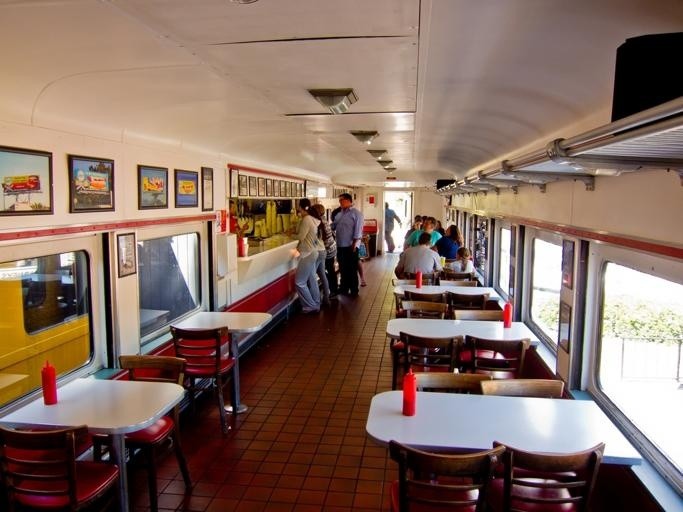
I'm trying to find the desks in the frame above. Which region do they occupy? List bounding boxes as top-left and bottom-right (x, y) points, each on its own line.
(174, 311), (273, 415)
(0, 376), (185, 511)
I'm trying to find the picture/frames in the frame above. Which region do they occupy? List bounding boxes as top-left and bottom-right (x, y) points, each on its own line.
(227, 168), (240, 199)
(199, 166), (214, 211)
(117, 232), (137, 278)
(136, 165), (168, 210)
(238, 175), (306, 200)
(0, 145), (54, 216)
(68, 154), (115, 213)
(174, 169), (198, 208)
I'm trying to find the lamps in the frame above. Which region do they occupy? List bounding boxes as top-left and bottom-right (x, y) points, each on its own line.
(367, 149), (388, 161)
(306, 88), (361, 117)
(383, 167), (397, 173)
(349, 130), (380, 146)
(376, 161), (394, 169)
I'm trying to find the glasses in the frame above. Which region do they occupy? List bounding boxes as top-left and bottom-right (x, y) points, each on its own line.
(339, 199), (344, 202)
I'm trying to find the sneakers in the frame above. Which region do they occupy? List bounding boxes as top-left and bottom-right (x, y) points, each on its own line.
(328, 292), (337, 298)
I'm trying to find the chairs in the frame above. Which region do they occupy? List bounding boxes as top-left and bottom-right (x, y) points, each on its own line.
(0, 424), (120, 511)
(92, 355), (194, 511)
(168, 324), (238, 435)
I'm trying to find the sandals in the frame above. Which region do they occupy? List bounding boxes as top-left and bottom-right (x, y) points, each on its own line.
(361, 280), (366, 287)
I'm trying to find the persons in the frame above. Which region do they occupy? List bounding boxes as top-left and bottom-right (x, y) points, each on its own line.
(286, 198), (321, 313)
(229, 200), (249, 240)
(395, 253), (475, 281)
(331, 206), (367, 287)
(309, 207), (331, 306)
(330, 193), (364, 301)
(385, 202), (403, 253)
(312, 204), (338, 300)
(402, 214), (471, 253)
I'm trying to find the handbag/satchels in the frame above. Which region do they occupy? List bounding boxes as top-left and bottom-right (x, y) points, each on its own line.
(358, 243), (366, 260)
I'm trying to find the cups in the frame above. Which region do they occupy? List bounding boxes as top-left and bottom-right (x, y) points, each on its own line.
(440, 257), (445, 268)
(254, 218), (267, 240)
(265, 200), (282, 235)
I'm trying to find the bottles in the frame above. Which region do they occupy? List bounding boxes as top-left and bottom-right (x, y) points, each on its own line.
(503, 302), (511, 328)
(415, 272), (421, 288)
(238, 234), (244, 257)
(401, 368), (416, 417)
(41, 360), (57, 406)
(243, 237), (248, 256)
